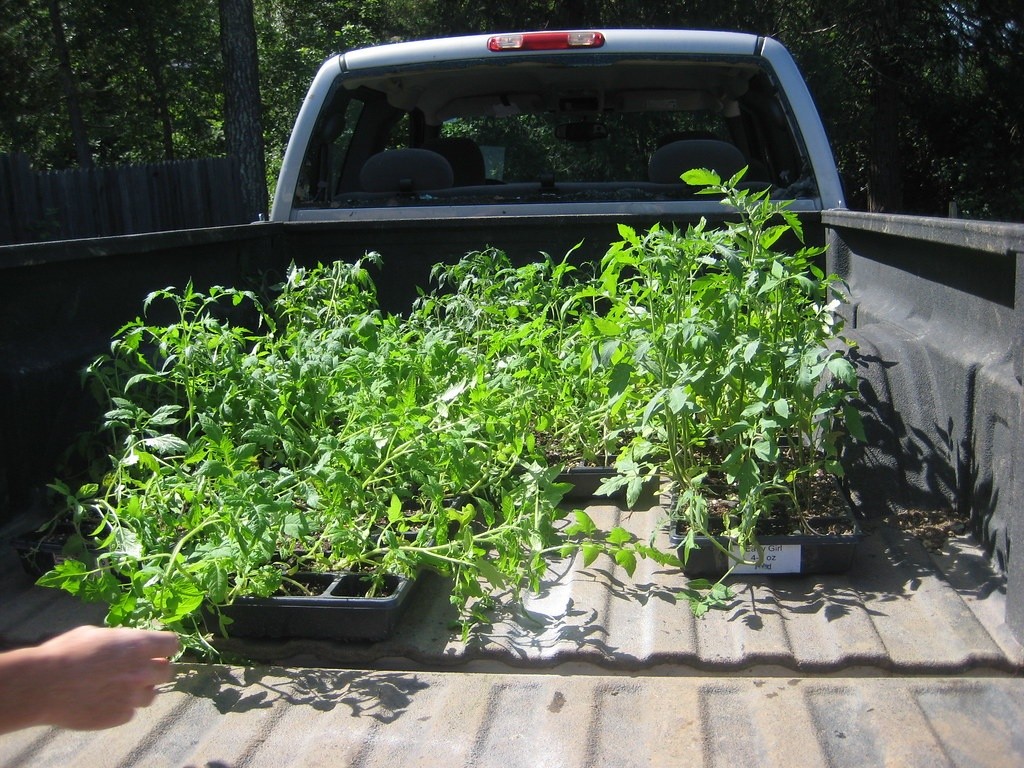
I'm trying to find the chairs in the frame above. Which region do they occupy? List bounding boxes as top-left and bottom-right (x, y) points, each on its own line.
(363, 149), (455, 195)
(419, 138), (506, 187)
(650, 138), (747, 185)
(657, 130), (715, 147)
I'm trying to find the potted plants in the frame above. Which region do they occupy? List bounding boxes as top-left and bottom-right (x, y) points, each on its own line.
(566, 165), (862, 574)
(24, 248), (686, 666)
(433, 249), (666, 501)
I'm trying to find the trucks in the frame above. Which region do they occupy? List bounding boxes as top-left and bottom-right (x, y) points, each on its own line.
(1, 28), (1024, 768)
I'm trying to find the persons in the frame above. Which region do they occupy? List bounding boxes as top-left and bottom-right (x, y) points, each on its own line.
(0, 625), (180, 735)
